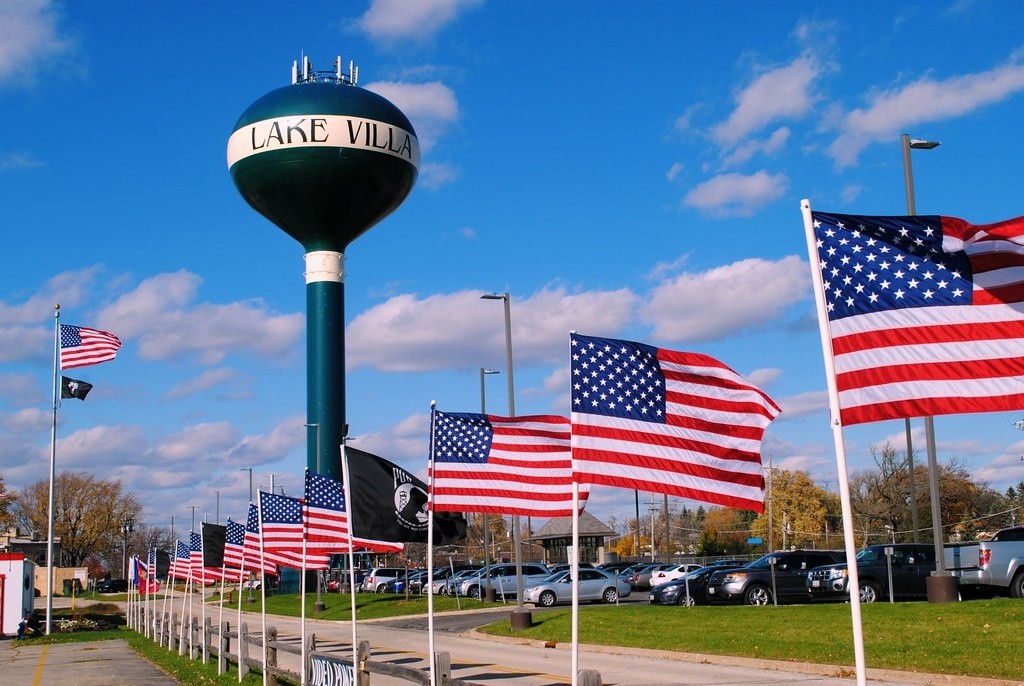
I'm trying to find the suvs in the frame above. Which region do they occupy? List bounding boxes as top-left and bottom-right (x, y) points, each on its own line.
(703, 548), (847, 608)
(804, 542), (951, 605)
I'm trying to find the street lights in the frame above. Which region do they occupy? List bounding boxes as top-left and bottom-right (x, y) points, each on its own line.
(238, 465), (252, 504)
(162, 514), (174, 550)
(901, 131), (962, 601)
(479, 367), (502, 587)
(208, 491), (222, 526)
(120, 523), (135, 579)
(481, 289), (533, 629)
(186, 505), (199, 533)
(302, 420), (322, 476)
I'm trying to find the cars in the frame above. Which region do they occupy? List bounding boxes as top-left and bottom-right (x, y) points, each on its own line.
(516, 567), (633, 607)
(648, 563), (705, 589)
(648, 565), (742, 610)
(386, 561), (552, 601)
(704, 558), (750, 568)
(325, 570), (364, 594)
(96, 579), (129, 593)
(360, 566), (413, 594)
(545, 560), (675, 590)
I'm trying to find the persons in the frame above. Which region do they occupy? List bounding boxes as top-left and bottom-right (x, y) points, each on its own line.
(354, 569), (365, 593)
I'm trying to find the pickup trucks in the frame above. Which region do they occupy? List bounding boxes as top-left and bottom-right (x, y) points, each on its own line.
(936, 523), (1024, 601)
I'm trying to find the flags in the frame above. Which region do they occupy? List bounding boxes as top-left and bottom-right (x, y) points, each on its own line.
(344, 446), (467, 546)
(260, 491), (361, 552)
(244, 504), (331, 571)
(60, 323), (123, 371)
(812, 210), (1024, 428)
(433, 410), (591, 518)
(61, 376), (93, 401)
(570, 332), (783, 515)
(130, 519), (278, 595)
(307, 472), (404, 554)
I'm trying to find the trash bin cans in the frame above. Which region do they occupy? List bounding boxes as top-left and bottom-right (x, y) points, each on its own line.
(604, 552), (617, 563)
(63, 578), (80, 596)
